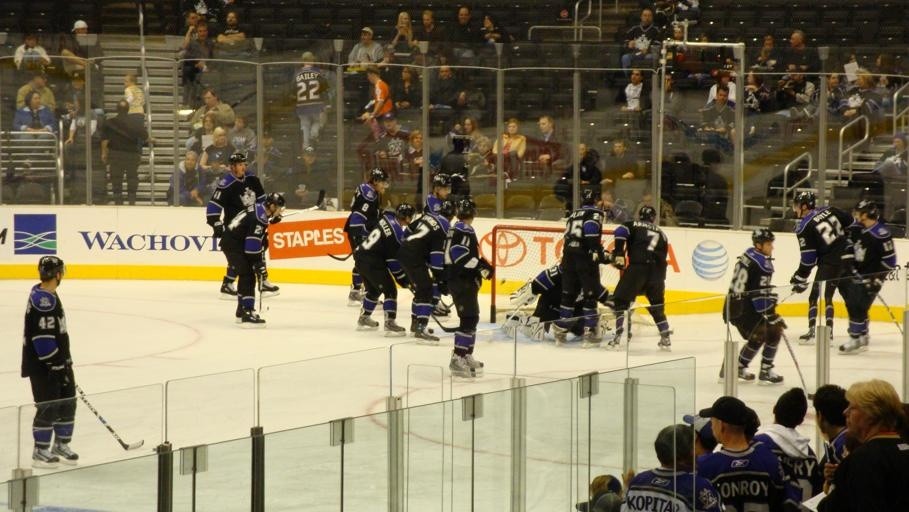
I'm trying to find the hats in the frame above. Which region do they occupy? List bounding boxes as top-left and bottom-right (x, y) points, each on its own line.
(773, 388), (807, 428)
(382, 112), (396, 122)
(302, 53), (316, 60)
(305, 146), (315, 155)
(699, 396), (748, 424)
(576, 489), (621, 512)
(71, 20), (88, 32)
(361, 27), (373, 34)
(744, 408), (761, 432)
(684, 414), (717, 448)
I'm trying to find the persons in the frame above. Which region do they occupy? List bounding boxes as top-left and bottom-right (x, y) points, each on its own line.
(825, 199), (899, 353)
(841, 52), (873, 86)
(623, 8), (665, 79)
(377, 111), (412, 169)
(652, 74), (684, 125)
(442, 9), (477, 62)
(779, 67), (816, 109)
(191, 113), (218, 156)
(477, 15), (514, 76)
(398, 208), (458, 343)
(442, 199), (493, 379)
(744, 68), (774, 112)
(401, 131), (433, 175)
(413, 11), (441, 44)
(344, 169), (390, 310)
(366, 67), (397, 137)
(705, 70), (739, 107)
(22, 256), (79, 469)
(425, 174), (454, 317)
(603, 142), (639, 189)
(15, 77), (56, 109)
(431, 64), (464, 108)
(781, 33), (822, 73)
(694, 395), (786, 510)
(169, 151), (209, 206)
(10, 93), (57, 170)
(63, 92), (98, 185)
(347, 26), (385, 78)
(661, 24), (691, 67)
(493, 118), (527, 183)
(290, 51), (329, 155)
(355, 202), (415, 338)
(874, 133), (909, 185)
(867, 51), (903, 88)
(624, 424), (725, 512)
(182, 21), (216, 108)
(247, 135), (283, 171)
(177, 11), (201, 35)
(289, 147), (329, 202)
(839, 72), (884, 127)
(220, 194), (285, 327)
(699, 87), (737, 138)
(528, 249), (613, 335)
(14, 31), (52, 82)
(585, 470), (634, 501)
(607, 204), (674, 349)
(751, 385), (822, 510)
(679, 411), (718, 462)
(790, 190), (867, 345)
(686, 34), (722, 74)
(544, 199), (607, 346)
(752, 35), (784, 74)
(813, 384), (851, 481)
(118, 72), (146, 151)
(190, 87), (236, 131)
(465, 137), (501, 178)
(388, 12), (418, 69)
(557, 145), (603, 206)
(817, 380), (909, 512)
(205, 154), (282, 295)
(218, 10), (252, 71)
(689, 150), (730, 225)
(618, 69), (654, 133)
(62, 19), (105, 108)
(530, 117), (565, 166)
(724, 229), (787, 383)
(100, 99), (155, 208)
(449, 117), (482, 155)
(62, 72), (97, 118)
(393, 65), (426, 111)
(226, 113), (257, 156)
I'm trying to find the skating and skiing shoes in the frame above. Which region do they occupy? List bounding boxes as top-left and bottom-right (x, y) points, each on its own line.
(830, 334), (834, 348)
(348, 290), (364, 306)
(258, 281), (279, 298)
(374, 300), (383, 310)
(435, 306), (451, 318)
(429, 310), (448, 321)
(658, 336), (672, 352)
(384, 321), (406, 337)
(32, 448), (60, 469)
(555, 334), (572, 347)
(357, 317), (380, 330)
(582, 335), (602, 349)
(837, 339), (862, 355)
(235, 306), (260, 323)
(860, 336), (869, 350)
(51, 442), (79, 465)
(220, 283), (239, 301)
(799, 331), (816, 345)
(411, 326), (434, 338)
(738, 367), (755, 383)
(450, 358), (475, 382)
(607, 335), (631, 351)
(242, 312), (266, 329)
(758, 371), (784, 385)
(415, 331), (439, 346)
(464, 355), (484, 378)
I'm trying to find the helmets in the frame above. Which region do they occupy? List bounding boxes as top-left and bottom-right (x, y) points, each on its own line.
(752, 229), (774, 243)
(229, 153), (247, 165)
(794, 192), (816, 209)
(433, 174), (452, 187)
(266, 192), (284, 208)
(39, 256), (63, 280)
(439, 201), (457, 217)
(855, 199), (880, 222)
(640, 206), (656, 222)
(583, 186), (601, 201)
(396, 204), (414, 218)
(370, 168), (387, 182)
(456, 199), (475, 218)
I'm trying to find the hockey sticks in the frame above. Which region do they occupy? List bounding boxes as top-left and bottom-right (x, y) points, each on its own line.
(875, 292), (904, 334)
(408, 284), (460, 333)
(259, 275), (270, 314)
(776, 290), (797, 306)
(439, 297), (455, 309)
(780, 326), (817, 400)
(75, 383), (145, 451)
(327, 251), (353, 261)
(267, 189), (326, 222)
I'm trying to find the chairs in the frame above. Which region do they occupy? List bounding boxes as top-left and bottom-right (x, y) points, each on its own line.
(154, 0), (909, 239)
(0, 0), (108, 205)
